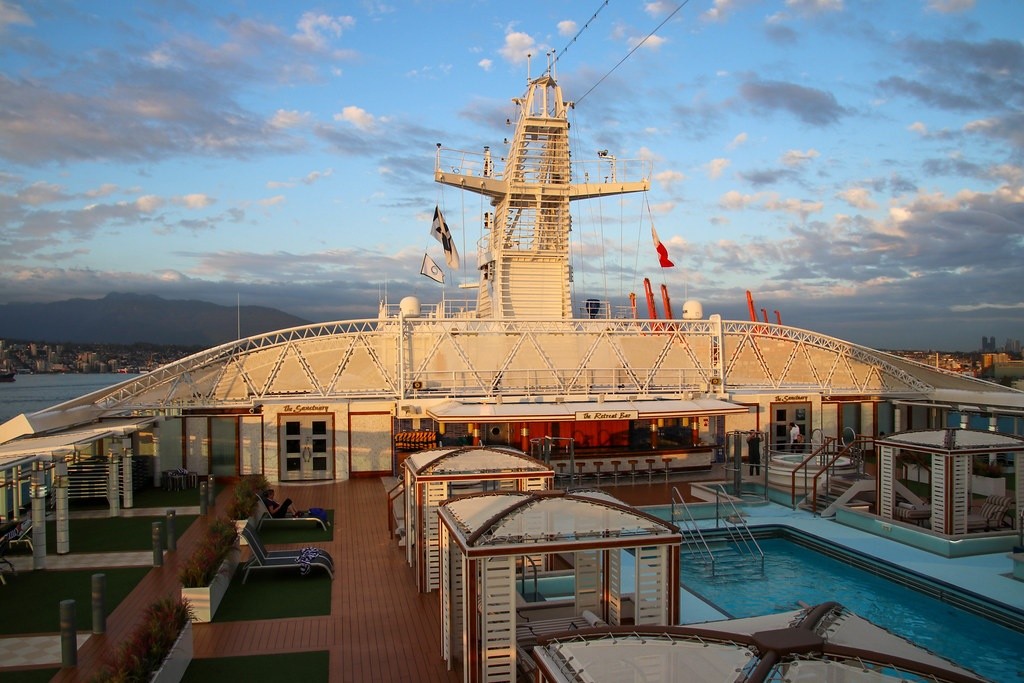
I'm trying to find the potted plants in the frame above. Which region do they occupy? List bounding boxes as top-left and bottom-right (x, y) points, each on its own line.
(227, 473), (273, 546)
(175, 512), (241, 623)
(86, 592), (193, 682)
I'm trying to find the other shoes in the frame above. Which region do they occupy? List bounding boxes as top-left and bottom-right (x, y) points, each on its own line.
(292, 511), (303, 518)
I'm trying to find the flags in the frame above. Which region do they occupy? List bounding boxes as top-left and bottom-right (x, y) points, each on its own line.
(430, 206), (461, 271)
(420, 253), (446, 285)
(651, 223), (675, 268)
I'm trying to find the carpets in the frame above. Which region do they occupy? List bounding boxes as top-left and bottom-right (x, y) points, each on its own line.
(0, 564), (155, 638)
(45, 475), (226, 511)
(0, 514), (199, 557)
(211, 561), (331, 622)
(0, 663), (62, 683)
(257, 508), (335, 544)
(180, 650), (329, 683)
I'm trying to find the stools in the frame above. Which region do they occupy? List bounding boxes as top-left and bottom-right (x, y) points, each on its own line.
(162, 470), (198, 491)
(593, 462), (604, 485)
(644, 459), (656, 482)
(661, 459), (672, 481)
(611, 460), (622, 484)
(628, 460), (639, 483)
(575, 462), (586, 486)
(556, 463), (566, 486)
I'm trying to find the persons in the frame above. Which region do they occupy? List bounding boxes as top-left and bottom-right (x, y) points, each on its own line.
(789, 422), (803, 453)
(264, 489), (304, 518)
(746, 430), (765, 476)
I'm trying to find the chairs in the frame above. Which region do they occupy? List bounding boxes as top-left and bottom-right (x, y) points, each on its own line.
(50, 455), (153, 509)
(491, 610), (611, 683)
(893, 495), (1014, 532)
(255, 489), (331, 532)
(241, 522), (335, 580)
(8, 525), (33, 552)
